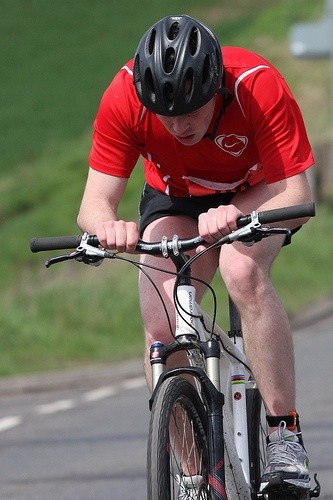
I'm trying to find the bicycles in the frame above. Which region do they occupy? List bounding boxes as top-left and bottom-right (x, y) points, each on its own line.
(30, 201), (321, 500)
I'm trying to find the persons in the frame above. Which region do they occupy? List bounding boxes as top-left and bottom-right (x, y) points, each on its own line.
(77, 15), (315, 500)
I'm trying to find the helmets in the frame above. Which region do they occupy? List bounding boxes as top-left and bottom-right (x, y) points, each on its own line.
(131, 15), (221, 117)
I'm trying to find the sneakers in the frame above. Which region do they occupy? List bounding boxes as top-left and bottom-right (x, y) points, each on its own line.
(260, 413), (322, 498)
(175, 465), (207, 500)
